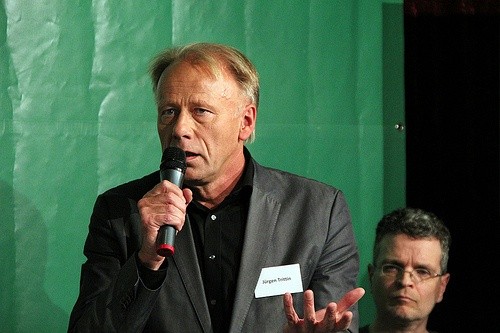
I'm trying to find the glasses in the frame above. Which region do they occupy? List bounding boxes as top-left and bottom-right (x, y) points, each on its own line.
(376, 263), (444, 282)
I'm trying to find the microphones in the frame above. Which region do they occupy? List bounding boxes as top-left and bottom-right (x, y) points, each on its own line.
(157, 147), (187, 257)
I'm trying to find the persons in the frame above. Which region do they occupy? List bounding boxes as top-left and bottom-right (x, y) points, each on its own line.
(358, 207), (452, 333)
(67, 40), (367, 333)
